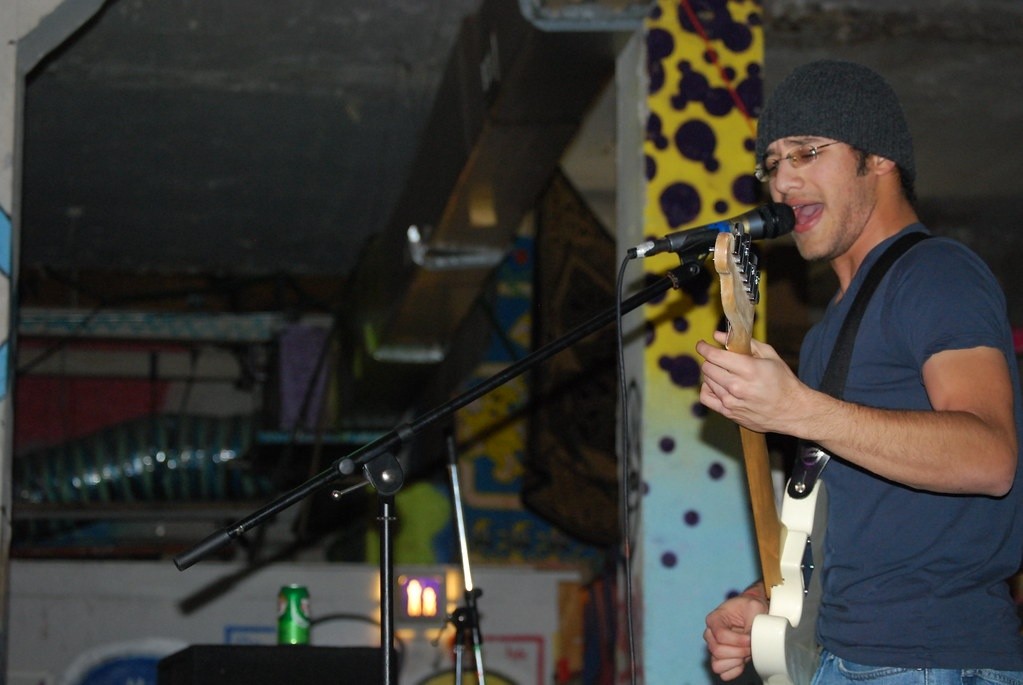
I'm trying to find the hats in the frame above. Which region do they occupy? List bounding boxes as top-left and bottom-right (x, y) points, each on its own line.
(754, 56), (917, 176)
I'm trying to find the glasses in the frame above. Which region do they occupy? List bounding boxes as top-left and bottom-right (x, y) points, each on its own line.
(757, 138), (842, 182)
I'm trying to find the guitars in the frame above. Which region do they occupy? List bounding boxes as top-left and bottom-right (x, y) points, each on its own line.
(710, 221), (829, 685)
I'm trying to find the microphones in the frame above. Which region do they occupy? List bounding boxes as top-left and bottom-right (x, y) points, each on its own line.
(626, 201), (794, 260)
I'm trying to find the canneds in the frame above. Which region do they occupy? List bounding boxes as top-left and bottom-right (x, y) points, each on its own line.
(277, 583), (311, 646)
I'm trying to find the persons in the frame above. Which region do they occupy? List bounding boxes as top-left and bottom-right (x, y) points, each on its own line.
(696, 60), (1023, 685)
